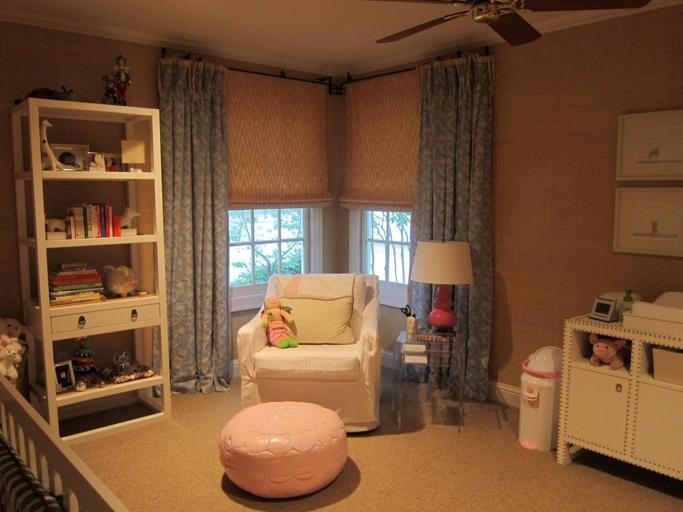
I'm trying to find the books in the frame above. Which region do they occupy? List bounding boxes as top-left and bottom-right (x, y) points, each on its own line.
(48, 260), (104, 305)
(66, 199), (122, 239)
(416, 332), (453, 342)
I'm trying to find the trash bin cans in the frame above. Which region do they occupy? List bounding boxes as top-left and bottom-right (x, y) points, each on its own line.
(519, 346), (571, 450)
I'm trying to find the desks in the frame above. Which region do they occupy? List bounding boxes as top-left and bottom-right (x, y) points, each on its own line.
(393, 331), (472, 433)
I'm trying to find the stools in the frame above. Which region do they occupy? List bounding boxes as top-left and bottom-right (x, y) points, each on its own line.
(220, 401), (348, 499)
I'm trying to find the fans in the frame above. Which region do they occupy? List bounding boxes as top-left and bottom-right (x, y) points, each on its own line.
(376, 0), (653, 47)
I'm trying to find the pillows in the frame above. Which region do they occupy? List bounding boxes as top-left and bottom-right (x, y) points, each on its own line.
(280, 296), (356, 345)
(278, 274), (366, 339)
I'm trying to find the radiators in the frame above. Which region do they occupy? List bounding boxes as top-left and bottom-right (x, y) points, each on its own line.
(0, 431), (64, 512)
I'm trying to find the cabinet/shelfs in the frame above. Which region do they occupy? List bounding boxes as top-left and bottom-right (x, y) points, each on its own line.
(556, 313), (683, 481)
(10, 97), (172, 442)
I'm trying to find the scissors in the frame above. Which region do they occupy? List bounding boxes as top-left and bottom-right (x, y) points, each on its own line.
(401, 305), (411, 317)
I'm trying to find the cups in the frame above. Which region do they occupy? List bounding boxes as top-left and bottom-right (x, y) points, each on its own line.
(406, 316), (419, 334)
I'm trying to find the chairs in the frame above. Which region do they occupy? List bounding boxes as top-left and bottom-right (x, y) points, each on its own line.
(236, 272), (381, 433)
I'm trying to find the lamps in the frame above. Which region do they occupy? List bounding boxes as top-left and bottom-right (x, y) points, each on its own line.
(410, 241), (473, 336)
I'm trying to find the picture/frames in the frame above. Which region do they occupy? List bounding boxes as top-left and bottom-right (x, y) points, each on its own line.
(612, 187), (683, 257)
(616, 109), (683, 181)
(54, 360), (75, 393)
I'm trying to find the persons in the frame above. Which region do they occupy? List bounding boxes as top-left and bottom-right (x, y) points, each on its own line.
(102, 78), (117, 104)
(112, 55), (132, 106)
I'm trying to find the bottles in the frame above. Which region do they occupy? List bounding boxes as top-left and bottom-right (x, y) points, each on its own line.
(620, 287), (635, 324)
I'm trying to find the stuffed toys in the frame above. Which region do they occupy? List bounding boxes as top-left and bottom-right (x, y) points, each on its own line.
(0, 317), (30, 341)
(0, 335), (23, 380)
(258, 296), (298, 347)
(585, 334), (627, 371)
